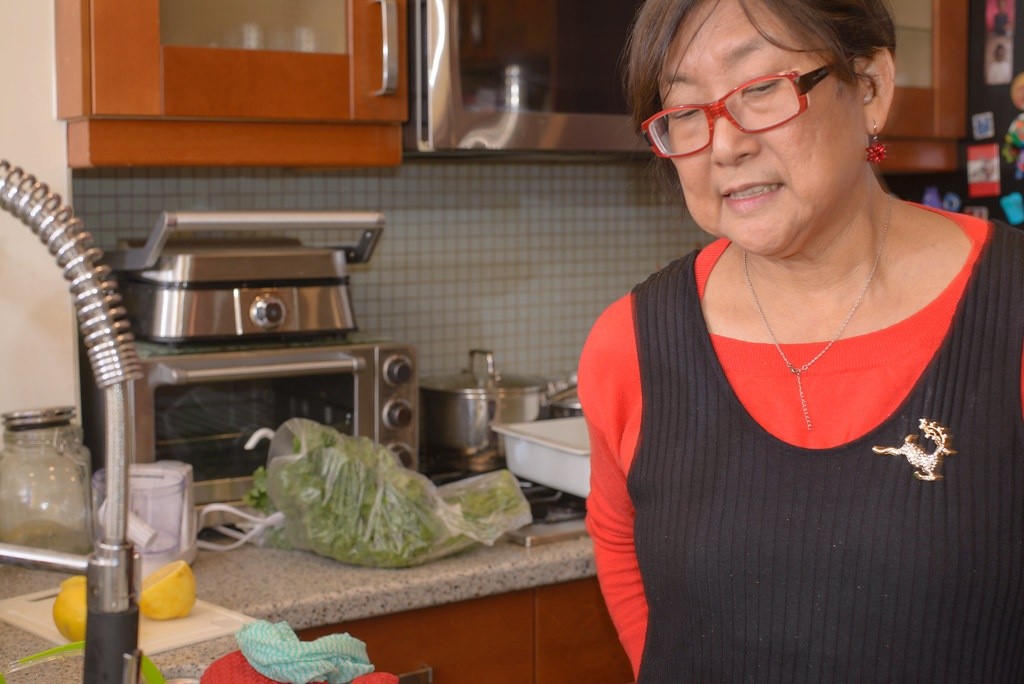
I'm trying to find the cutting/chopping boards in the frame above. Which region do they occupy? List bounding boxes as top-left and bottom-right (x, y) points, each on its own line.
(1, 589), (260, 656)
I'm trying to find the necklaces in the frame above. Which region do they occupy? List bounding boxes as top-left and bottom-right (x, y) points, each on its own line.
(739, 193), (892, 433)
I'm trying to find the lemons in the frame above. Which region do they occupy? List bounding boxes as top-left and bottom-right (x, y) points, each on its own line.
(51, 559), (194, 641)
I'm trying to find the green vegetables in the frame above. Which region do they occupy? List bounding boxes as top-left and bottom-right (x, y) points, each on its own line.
(243, 426), (525, 568)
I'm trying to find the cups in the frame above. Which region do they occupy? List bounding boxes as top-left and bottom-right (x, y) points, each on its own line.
(95, 462), (185, 581)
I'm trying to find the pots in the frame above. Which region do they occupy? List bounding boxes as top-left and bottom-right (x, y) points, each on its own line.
(551, 376), (584, 417)
(420, 348), (548, 451)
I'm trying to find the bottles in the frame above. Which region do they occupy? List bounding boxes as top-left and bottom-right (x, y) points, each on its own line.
(0, 405), (94, 555)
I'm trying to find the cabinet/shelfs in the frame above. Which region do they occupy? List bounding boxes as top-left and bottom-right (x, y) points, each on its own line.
(292, 575), (632, 684)
(51, 0), (410, 170)
(867, 1), (966, 170)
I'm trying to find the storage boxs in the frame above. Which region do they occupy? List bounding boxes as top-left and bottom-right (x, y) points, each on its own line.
(490, 412), (592, 498)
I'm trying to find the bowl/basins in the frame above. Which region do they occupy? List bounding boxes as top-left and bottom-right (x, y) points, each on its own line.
(0, 641), (167, 683)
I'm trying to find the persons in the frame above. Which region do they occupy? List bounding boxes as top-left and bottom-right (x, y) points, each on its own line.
(573, 0), (1024, 684)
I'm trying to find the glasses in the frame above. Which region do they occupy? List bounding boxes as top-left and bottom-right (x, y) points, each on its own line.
(641, 36), (878, 159)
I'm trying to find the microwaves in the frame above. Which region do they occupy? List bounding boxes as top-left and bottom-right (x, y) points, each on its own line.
(403, 0), (659, 157)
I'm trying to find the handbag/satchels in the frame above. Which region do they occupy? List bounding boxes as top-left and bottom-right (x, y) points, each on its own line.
(193, 415), (536, 571)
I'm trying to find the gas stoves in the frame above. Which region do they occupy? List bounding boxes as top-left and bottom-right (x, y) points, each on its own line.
(421, 449), (590, 549)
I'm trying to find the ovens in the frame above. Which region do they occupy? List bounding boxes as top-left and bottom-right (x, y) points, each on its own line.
(81, 210), (420, 533)
